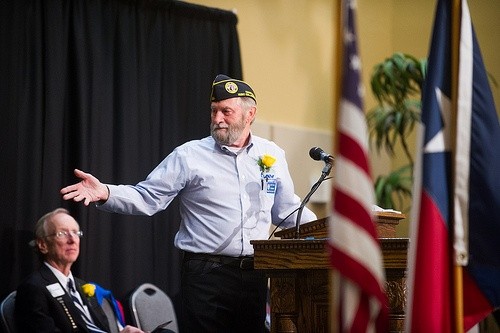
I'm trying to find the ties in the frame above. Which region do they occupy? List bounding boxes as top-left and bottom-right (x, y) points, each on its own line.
(67, 277), (109, 333)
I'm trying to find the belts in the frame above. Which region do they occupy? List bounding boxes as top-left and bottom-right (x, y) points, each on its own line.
(180, 249), (256, 270)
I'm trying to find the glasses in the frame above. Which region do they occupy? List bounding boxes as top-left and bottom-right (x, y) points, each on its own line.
(40, 229), (83, 240)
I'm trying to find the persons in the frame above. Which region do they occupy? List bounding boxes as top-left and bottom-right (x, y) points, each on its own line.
(13, 209), (152, 333)
(60, 74), (319, 333)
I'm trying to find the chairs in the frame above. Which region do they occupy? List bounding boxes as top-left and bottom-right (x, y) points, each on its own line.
(130, 282), (178, 333)
(2, 291), (18, 333)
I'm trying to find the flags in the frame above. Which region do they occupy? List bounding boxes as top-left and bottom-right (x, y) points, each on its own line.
(405, 0), (500, 333)
(328, 0), (388, 333)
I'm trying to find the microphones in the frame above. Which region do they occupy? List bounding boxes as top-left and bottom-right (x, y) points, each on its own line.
(309, 147), (334, 163)
(68, 279), (75, 293)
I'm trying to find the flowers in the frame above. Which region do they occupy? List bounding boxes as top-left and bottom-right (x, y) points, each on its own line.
(257, 155), (276, 171)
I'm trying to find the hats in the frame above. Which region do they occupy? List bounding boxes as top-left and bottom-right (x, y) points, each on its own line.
(210, 74), (258, 102)
